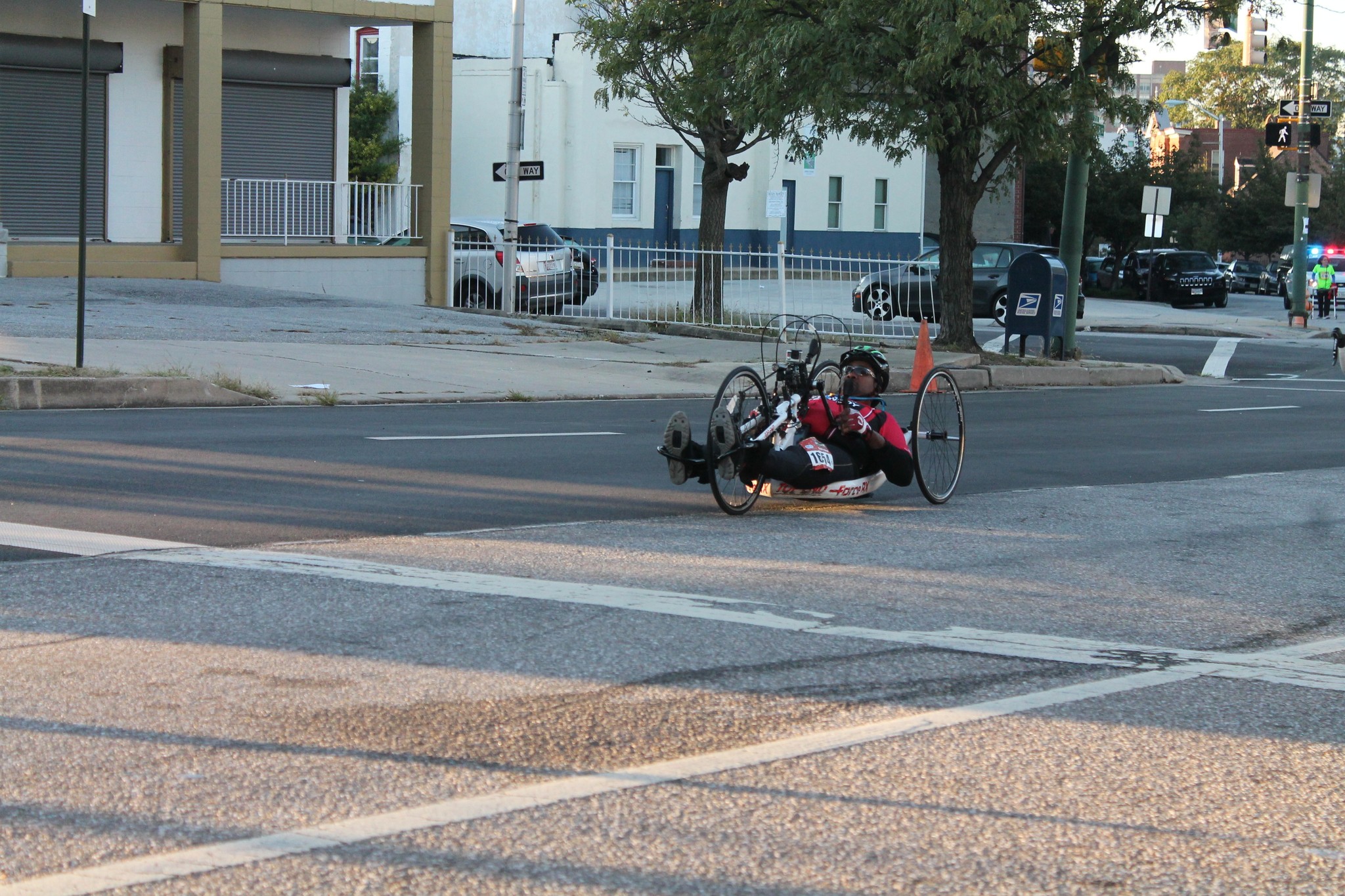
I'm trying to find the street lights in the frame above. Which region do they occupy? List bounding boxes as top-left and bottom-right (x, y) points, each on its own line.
(1165, 99), (1224, 263)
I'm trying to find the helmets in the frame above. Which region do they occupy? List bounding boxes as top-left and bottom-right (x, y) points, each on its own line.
(840, 345), (890, 393)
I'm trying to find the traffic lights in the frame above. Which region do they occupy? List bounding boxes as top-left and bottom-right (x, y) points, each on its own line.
(1265, 123), (1291, 146)
(1204, 10), (1231, 51)
(1240, 14), (1269, 65)
(1308, 123), (1321, 148)
(1097, 42), (1119, 78)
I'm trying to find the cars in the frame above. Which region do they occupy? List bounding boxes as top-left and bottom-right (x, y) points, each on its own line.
(1222, 259), (1266, 293)
(1214, 263), (1231, 274)
(1284, 248), (1345, 309)
(1083, 254), (1128, 292)
(1256, 260), (1279, 296)
(528, 235), (601, 316)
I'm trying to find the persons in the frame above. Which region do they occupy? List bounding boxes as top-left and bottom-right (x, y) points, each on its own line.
(663, 345), (914, 487)
(1312, 255), (1335, 319)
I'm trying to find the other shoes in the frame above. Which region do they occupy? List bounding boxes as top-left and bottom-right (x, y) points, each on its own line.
(1324, 316), (1330, 319)
(664, 410), (692, 485)
(711, 406), (742, 481)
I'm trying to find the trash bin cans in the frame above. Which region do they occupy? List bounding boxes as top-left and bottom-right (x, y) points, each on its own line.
(1004, 251), (1069, 360)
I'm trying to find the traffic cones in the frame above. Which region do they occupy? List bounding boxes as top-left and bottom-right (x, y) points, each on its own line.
(897, 319), (948, 393)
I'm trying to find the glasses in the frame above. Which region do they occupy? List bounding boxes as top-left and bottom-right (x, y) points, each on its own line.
(841, 365), (877, 379)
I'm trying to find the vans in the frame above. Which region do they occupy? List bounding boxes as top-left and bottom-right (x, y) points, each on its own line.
(1275, 244), (1322, 296)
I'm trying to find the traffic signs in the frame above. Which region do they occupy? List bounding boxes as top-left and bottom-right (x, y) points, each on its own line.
(1278, 100), (1332, 118)
(492, 160), (545, 183)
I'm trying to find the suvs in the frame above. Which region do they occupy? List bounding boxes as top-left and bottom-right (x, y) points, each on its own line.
(376, 219), (579, 314)
(1152, 250), (1228, 309)
(849, 241), (1087, 321)
(1122, 248), (1180, 299)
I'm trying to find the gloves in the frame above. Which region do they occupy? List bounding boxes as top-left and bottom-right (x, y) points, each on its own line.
(834, 408), (872, 441)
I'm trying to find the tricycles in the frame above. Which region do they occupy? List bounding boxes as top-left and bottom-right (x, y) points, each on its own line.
(655, 312), (969, 517)
